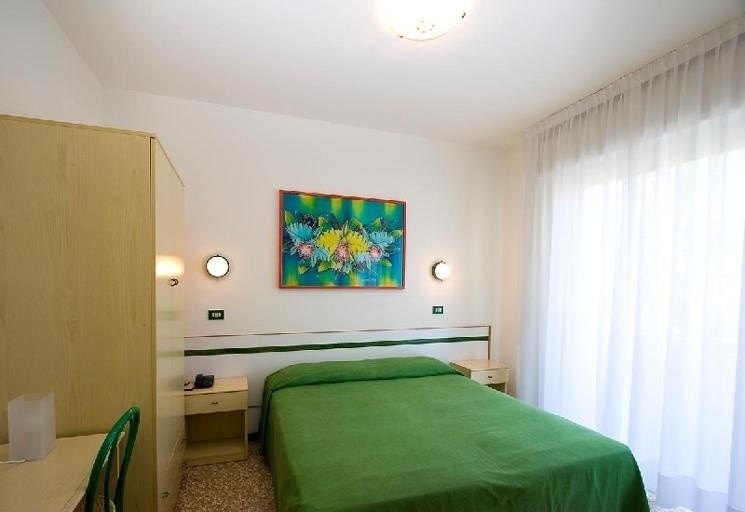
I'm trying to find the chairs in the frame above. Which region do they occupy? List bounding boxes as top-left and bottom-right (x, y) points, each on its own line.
(84, 407), (142, 511)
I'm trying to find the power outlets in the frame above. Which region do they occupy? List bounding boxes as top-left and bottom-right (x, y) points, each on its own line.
(432, 306), (443, 313)
(208, 309), (225, 320)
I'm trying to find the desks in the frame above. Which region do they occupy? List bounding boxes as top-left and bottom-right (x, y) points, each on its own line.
(1, 431), (126, 511)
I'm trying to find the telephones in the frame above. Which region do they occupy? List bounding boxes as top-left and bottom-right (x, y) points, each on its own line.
(194, 374), (214, 389)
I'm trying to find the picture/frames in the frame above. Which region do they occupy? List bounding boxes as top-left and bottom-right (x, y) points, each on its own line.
(278, 189), (406, 290)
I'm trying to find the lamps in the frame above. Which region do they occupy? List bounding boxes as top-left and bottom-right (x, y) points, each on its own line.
(205, 254), (230, 279)
(432, 260), (452, 282)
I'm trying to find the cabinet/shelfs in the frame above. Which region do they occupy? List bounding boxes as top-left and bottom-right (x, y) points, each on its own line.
(1, 112), (187, 511)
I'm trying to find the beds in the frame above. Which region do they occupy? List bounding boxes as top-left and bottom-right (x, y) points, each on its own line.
(257, 357), (652, 511)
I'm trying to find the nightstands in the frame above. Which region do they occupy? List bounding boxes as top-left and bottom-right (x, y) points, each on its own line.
(183, 376), (249, 467)
(450, 359), (510, 394)
